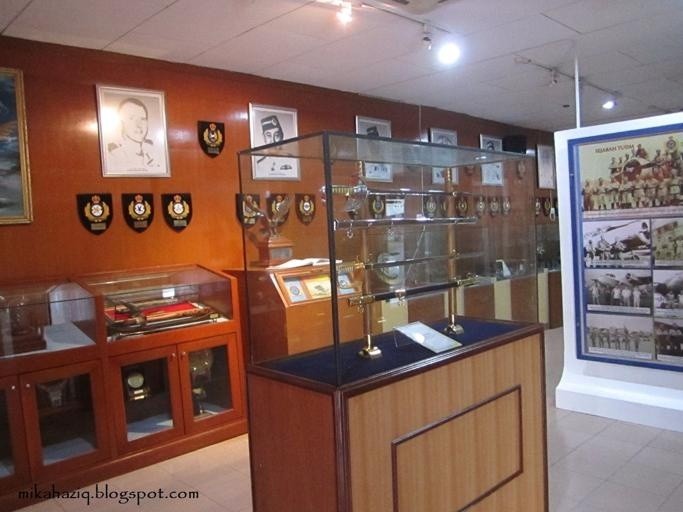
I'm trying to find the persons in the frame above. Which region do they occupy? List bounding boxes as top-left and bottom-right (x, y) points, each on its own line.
(256, 116), (292, 173)
(106, 97), (164, 173)
(578, 148), (683, 363)
(362, 126), (388, 177)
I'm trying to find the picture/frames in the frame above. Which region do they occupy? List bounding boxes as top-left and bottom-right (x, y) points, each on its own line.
(353, 113), (557, 190)
(0, 66), (36, 226)
(93, 83), (171, 178)
(247, 100), (303, 183)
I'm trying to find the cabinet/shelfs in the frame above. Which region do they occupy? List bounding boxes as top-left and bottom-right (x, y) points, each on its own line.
(1, 260), (249, 512)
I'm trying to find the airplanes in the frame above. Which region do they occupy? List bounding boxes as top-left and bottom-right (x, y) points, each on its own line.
(591, 272), (652, 298)
(585, 220), (650, 253)
(611, 135), (683, 185)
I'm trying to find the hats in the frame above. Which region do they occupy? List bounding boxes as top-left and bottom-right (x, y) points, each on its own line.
(261, 116), (279, 130)
